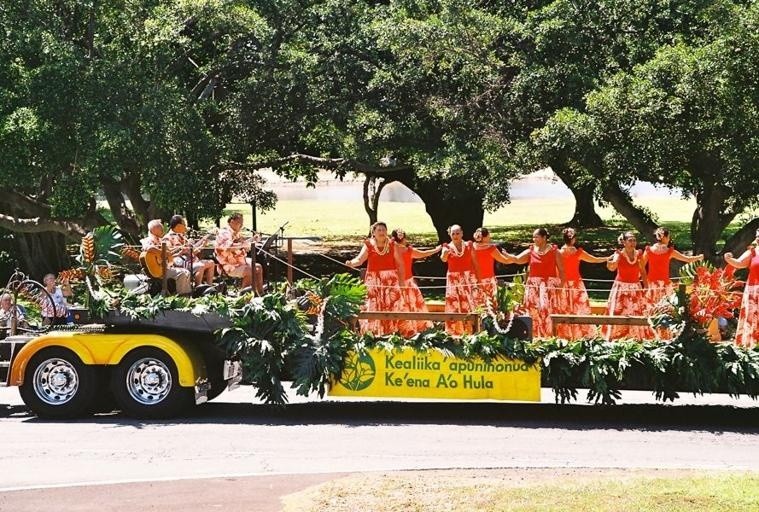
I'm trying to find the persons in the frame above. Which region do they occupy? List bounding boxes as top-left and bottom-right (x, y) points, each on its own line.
(214, 212), (265, 296)
(39, 272), (74, 326)
(0, 292), (28, 336)
(162, 215), (216, 287)
(138, 218), (193, 296)
(346, 223), (704, 340)
(724, 227), (759, 349)
